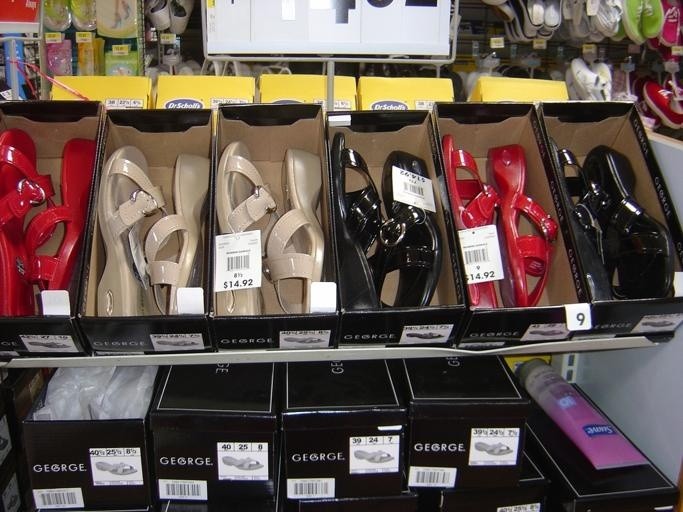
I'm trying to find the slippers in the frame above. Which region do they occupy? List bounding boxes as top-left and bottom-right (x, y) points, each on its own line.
(484, 0), (683, 45)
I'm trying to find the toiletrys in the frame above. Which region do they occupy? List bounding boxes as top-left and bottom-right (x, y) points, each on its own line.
(514, 355), (654, 474)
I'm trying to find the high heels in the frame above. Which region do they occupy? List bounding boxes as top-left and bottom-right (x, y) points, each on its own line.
(112, 4), (132, 28)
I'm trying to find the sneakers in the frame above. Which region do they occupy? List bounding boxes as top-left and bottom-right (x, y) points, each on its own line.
(142, 0), (194, 34)
(565, 57), (683, 130)
